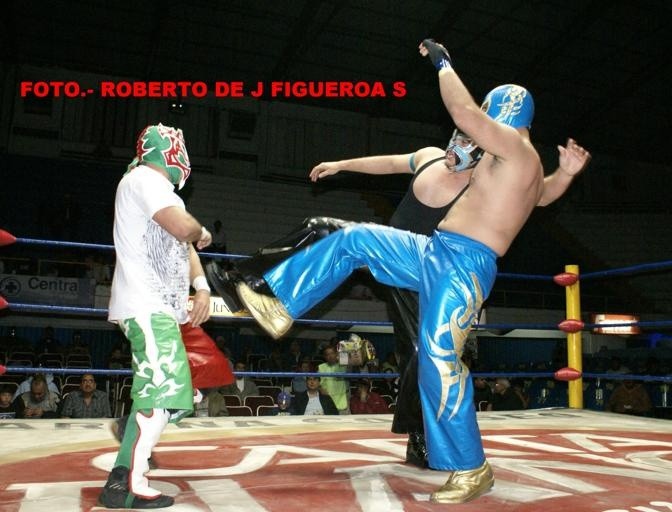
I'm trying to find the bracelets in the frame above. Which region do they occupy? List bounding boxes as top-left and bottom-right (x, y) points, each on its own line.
(191, 274), (212, 291)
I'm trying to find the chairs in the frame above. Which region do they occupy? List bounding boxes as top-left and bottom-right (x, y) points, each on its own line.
(0, 340), (672, 426)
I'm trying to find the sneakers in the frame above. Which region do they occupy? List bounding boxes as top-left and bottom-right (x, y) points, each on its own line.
(236, 281), (293, 339)
(429, 461), (495, 504)
(406, 432), (433, 470)
(205, 260), (246, 312)
(99, 472), (173, 508)
(111, 415), (157, 470)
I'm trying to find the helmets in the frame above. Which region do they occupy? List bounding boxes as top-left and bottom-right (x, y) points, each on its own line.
(480, 84), (535, 129)
(124, 122), (192, 191)
(445, 129), (484, 172)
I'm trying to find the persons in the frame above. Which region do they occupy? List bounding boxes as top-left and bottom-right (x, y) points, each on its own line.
(1, 219), (640, 312)
(205, 128), (593, 472)
(0, 326), (672, 418)
(237, 35), (546, 504)
(96, 119), (216, 509)
